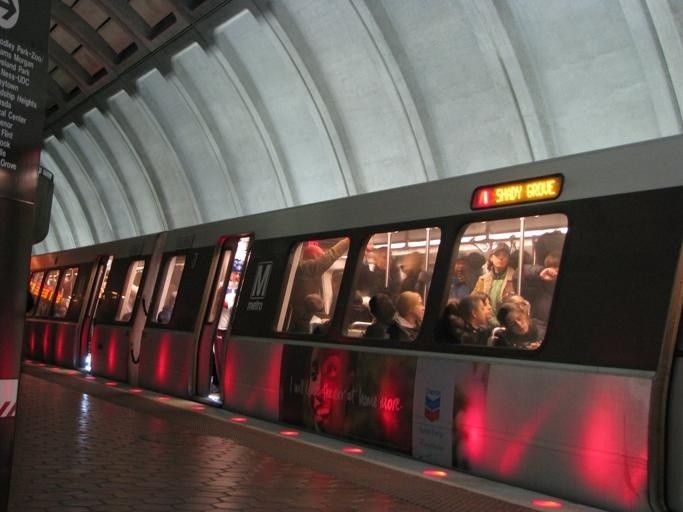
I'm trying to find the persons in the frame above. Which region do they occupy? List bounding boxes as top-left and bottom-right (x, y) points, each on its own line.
(301, 345), (356, 437)
(211, 259), (244, 391)
(156, 291), (178, 324)
(287, 230), (562, 351)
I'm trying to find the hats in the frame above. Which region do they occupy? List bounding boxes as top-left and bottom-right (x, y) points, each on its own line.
(492, 243), (511, 255)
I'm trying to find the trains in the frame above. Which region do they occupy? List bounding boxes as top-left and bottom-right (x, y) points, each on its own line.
(24, 129), (683, 509)
